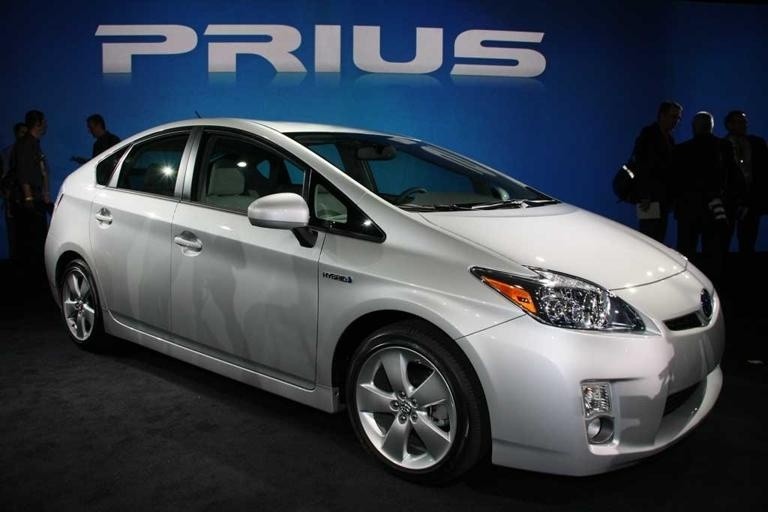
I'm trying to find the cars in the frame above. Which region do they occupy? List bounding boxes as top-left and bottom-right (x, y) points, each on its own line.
(39, 116), (730, 487)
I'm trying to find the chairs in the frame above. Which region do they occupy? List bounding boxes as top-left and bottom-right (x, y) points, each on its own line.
(143, 162), (172, 195)
(312, 184), (347, 224)
(201, 160), (260, 214)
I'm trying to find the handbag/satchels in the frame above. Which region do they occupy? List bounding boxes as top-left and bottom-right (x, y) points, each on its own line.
(611, 161), (644, 205)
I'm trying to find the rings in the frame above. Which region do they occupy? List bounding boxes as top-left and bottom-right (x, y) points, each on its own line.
(47, 199), (50, 202)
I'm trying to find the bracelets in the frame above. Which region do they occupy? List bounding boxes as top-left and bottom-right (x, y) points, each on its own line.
(40, 192), (49, 195)
(24, 195), (36, 202)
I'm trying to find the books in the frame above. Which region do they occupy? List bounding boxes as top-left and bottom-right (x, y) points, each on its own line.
(635, 201), (660, 221)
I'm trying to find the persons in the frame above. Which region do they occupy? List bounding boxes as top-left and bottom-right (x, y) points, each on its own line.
(0, 122), (50, 267)
(724, 110), (767, 256)
(8, 110), (50, 306)
(675, 110), (752, 272)
(627, 100), (684, 245)
(68, 113), (123, 166)
